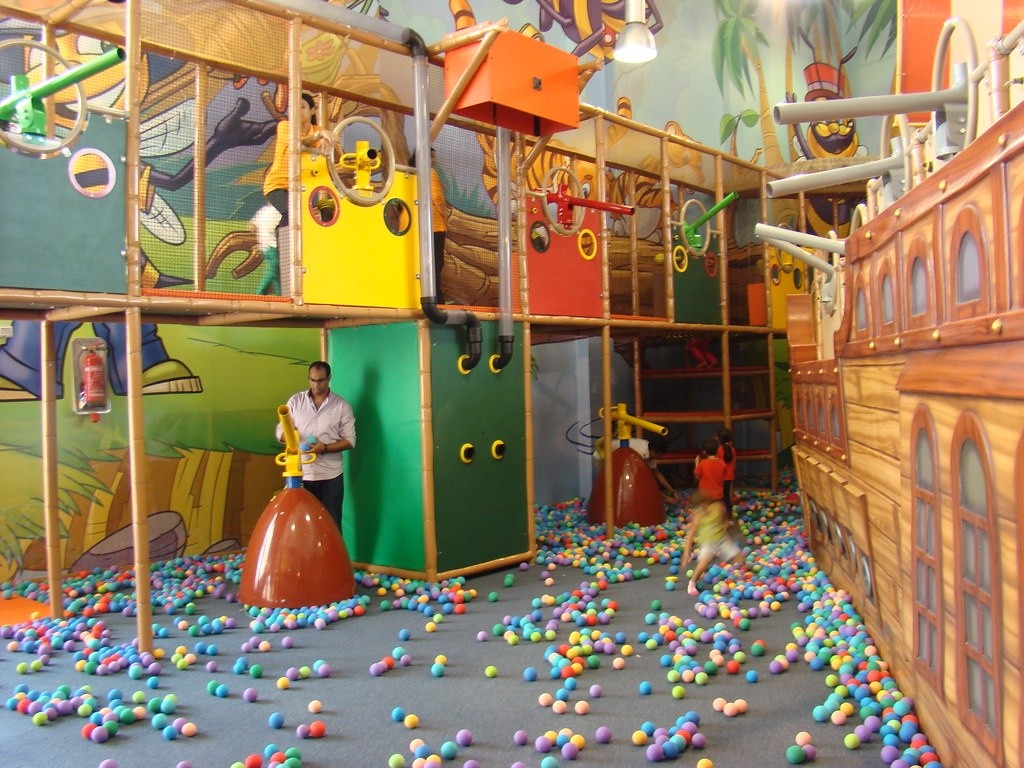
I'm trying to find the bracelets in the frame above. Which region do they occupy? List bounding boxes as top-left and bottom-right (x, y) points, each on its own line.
(321, 444), (327, 456)
(315, 131), (323, 140)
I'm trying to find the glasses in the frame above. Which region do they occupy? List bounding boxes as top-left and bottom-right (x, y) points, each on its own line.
(308, 375), (327, 382)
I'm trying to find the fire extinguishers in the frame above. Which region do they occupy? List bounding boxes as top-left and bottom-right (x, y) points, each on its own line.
(76, 342), (107, 410)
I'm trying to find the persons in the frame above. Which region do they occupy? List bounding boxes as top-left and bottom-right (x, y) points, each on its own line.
(682, 336), (719, 370)
(679, 476), (744, 597)
(275, 361), (357, 542)
(692, 427), (734, 518)
(408, 145), (452, 304)
(250, 93), (341, 296)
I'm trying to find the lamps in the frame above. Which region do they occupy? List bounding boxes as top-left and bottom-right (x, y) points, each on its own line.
(614, 0), (656, 64)
